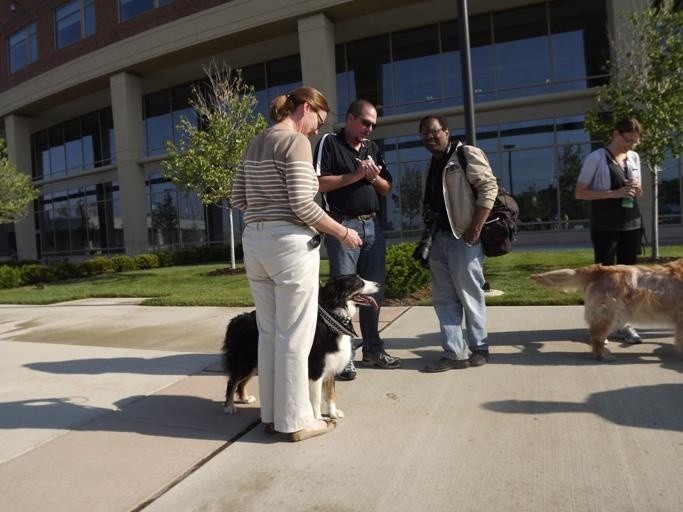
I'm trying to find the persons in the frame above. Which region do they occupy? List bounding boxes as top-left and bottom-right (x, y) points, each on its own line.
(312, 98), (401, 380)
(418, 114), (499, 372)
(341, 226), (348, 243)
(574, 119), (643, 344)
(227, 85), (363, 441)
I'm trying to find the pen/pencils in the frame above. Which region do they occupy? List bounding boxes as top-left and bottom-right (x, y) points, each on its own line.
(355, 158), (365, 163)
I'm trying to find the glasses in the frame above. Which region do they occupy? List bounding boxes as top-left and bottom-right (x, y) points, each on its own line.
(621, 134), (639, 145)
(352, 113), (377, 130)
(318, 112), (324, 130)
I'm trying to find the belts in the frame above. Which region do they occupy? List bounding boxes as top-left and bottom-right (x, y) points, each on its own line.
(344, 211), (379, 221)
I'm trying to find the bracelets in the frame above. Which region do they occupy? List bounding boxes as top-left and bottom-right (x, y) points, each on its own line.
(640, 187), (643, 198)
(367, 178), (377, 185)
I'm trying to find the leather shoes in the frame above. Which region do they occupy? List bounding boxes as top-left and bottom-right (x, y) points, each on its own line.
(290, 418), (337, 442)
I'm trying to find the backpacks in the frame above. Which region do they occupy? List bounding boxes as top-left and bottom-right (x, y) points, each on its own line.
(456, 141), (521, 258)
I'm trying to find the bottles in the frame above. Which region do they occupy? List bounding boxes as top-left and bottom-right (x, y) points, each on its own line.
(621, 168), (641, 209)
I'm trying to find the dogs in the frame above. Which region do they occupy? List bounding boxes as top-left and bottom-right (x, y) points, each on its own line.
(529, 256), (683, 363)
(222, 272), (382, 421)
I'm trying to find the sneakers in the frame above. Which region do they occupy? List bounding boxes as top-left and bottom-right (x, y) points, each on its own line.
(615, 324), (643, 344)
(361, 350), (402, 369)
(470, 348), (489, 366)
(337, 346), (357, 381)
(603, 338), (609, 344)
(423, 354), (470, 372)
